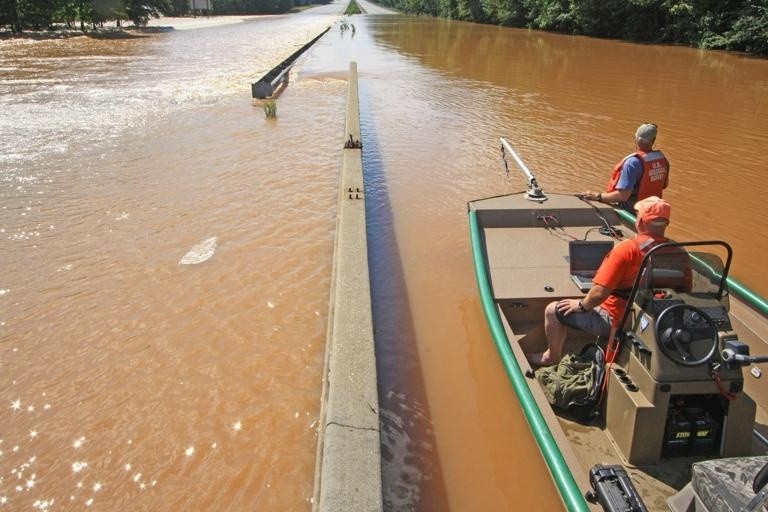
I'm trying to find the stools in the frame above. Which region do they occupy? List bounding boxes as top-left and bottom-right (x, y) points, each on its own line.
(666, 454), (767, 511)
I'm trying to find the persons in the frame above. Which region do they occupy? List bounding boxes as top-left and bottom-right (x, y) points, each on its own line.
(525, 196), (692, 366)
(581, 124), (669, 217)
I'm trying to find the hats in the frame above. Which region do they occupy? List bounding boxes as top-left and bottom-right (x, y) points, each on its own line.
(634, 195), (671, 226)
(636, 124), (657, 142)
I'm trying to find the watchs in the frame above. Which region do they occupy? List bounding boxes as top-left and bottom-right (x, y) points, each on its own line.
(596, 193), (602, 202)
(579, 301), (588, 312)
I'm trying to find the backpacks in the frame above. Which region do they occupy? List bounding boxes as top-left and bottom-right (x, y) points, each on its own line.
(536, 343), (605, 410)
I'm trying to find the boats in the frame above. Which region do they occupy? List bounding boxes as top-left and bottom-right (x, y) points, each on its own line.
(467, 136), (768, 512)
